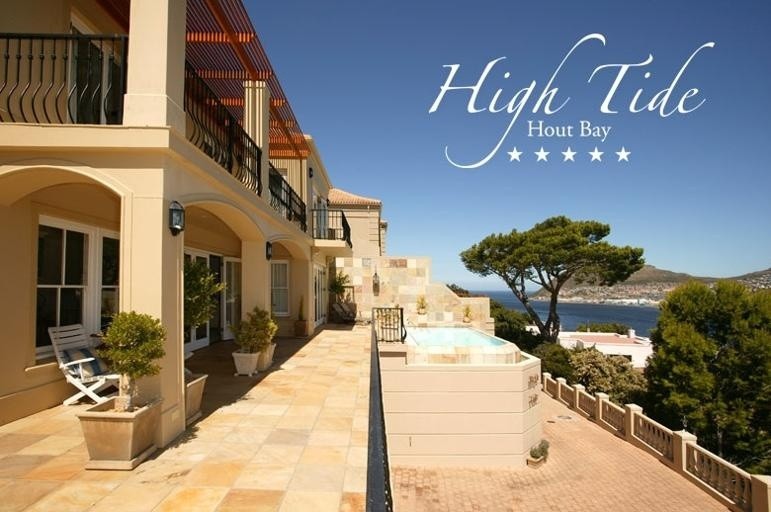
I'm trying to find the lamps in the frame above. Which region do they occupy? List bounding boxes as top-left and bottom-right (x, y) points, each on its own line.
(169, 200), (186, 236)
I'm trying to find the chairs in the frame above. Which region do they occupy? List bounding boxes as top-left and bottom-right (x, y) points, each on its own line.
(48, 324), (120, 406)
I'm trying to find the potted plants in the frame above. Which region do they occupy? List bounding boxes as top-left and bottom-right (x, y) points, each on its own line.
(227, 305), (280, 377)
(416, 295), (429, 325)
(527, 440), (551, 469)
(183, 253), (228, 427)
(75, 310), (168, 470)
(462, 304), (472, 323)
